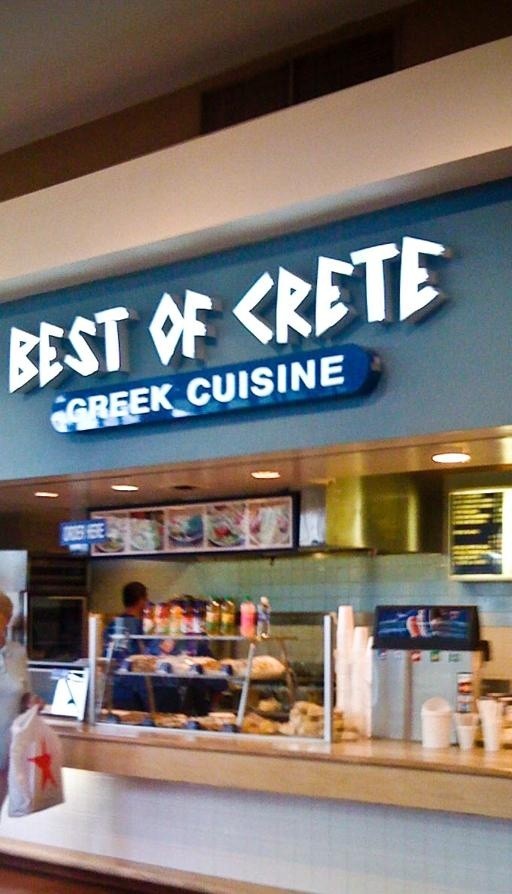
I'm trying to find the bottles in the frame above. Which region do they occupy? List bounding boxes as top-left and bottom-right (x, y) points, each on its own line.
(141, 594), (270, 637)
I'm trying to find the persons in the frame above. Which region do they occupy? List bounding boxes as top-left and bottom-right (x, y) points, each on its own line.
(134, 593), (230, 718)
(103, 579), (151, 714)
(0, 587), (47, 809)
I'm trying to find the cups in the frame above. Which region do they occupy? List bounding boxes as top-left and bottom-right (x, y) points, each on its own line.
(453, 700), (504, 754)
(335, 604), (373, 738)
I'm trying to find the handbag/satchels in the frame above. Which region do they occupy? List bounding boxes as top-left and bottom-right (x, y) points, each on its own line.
(6, 704), (66, 819)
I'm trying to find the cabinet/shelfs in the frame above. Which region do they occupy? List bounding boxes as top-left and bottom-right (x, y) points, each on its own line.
(94, 634), (298, 732)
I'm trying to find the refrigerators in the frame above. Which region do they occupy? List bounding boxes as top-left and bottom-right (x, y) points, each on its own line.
(27, 555), (91, 664)
